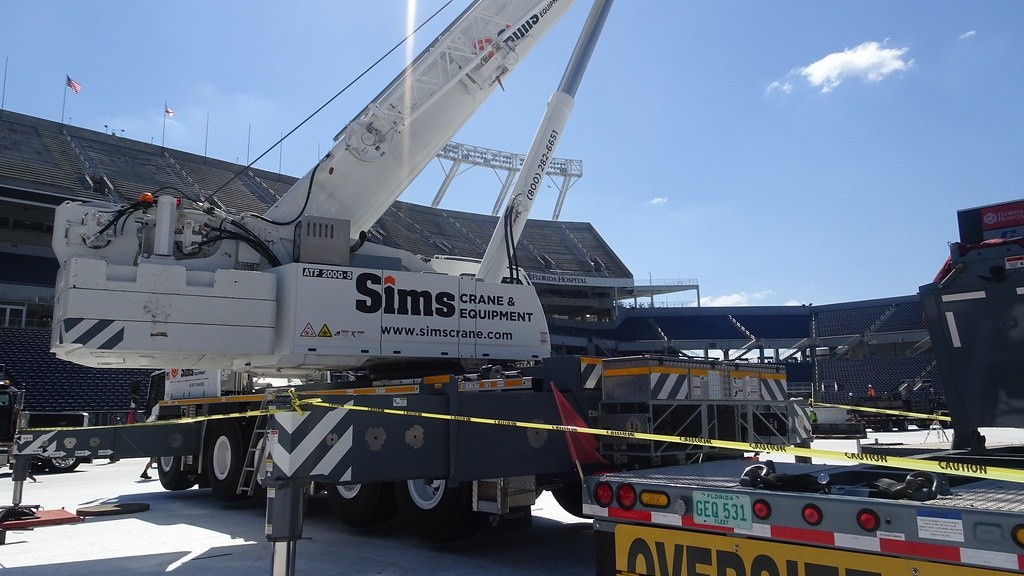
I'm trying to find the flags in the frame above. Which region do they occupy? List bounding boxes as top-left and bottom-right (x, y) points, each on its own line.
(551, 380), (608, 464)
(67, 75), (82, 93)
(165, 105), (174, 118)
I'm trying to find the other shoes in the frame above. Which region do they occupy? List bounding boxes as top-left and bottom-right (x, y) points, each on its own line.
(140, 470), (152, 479)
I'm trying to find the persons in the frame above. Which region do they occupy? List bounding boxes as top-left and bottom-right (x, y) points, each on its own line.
(868, 385), (875, 397)
(140, 396), (164, 480)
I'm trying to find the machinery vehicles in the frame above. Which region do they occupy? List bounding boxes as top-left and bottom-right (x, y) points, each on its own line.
(50, 0), (815, 546)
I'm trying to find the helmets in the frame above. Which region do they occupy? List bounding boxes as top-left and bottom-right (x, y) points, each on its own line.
(140, 192), (154, 203)
(867, 385), (872, 388)
(849, 393), (853, 396)
